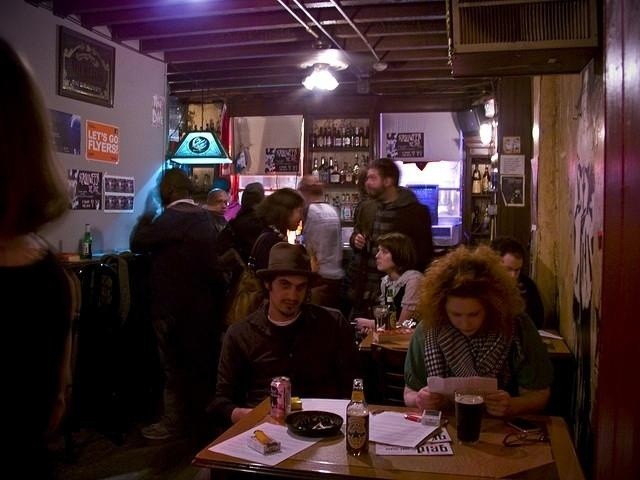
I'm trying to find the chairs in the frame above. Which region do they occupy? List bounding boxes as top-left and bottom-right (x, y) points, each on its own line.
(371, 344), (407, 407)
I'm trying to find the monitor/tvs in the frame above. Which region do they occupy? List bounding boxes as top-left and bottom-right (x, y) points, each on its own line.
(457, 105), (490, 136)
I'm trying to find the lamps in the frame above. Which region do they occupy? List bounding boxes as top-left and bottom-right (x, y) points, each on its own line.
(299, 37), (350, 91)
(169, 90), (234, 166)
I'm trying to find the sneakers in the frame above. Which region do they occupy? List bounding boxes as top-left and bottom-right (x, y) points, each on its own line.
(139, 416), (187, 440)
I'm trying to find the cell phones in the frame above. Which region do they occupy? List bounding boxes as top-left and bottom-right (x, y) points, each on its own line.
(508, 418), (541, 432)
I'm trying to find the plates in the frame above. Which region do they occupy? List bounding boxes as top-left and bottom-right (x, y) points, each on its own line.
(281, 411), (344, 435)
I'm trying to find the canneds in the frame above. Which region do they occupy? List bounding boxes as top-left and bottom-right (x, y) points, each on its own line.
(270, 376), (291, 417)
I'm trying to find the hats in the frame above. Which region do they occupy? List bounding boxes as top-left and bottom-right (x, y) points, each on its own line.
(255, 242), (323, 289)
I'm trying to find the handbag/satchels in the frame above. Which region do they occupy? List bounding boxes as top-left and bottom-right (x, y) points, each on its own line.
(224, 269), (265, 328)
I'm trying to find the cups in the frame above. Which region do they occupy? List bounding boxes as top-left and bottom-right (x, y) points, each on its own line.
(453, 389), (487, 446)
(375, 304), (389, 332)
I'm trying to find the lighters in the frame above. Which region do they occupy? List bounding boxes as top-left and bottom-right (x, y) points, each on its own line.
(254, 430), (270, 443)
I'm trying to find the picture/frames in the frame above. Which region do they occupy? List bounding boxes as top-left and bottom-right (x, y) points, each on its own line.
(500, 174), (526, 208)
(55, 24), (116, 109)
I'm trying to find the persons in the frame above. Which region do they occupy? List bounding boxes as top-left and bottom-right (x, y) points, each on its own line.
(0, 37), (82, 480)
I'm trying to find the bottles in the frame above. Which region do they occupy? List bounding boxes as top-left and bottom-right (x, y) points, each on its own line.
(385, 287), (399, 328)
(186, 117), (222, 140)
(346, 380), (370, 458)
(473, 163), (496, 234)
(309, 122), (368, 222)
(187, 174), (214, 195)
(82, 222), (94, 258)
(264, 147), (277, 172)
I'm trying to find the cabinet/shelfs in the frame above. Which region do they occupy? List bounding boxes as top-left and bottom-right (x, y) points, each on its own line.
(466, 155), (499, 248)
(303, 111), (381, 228)
